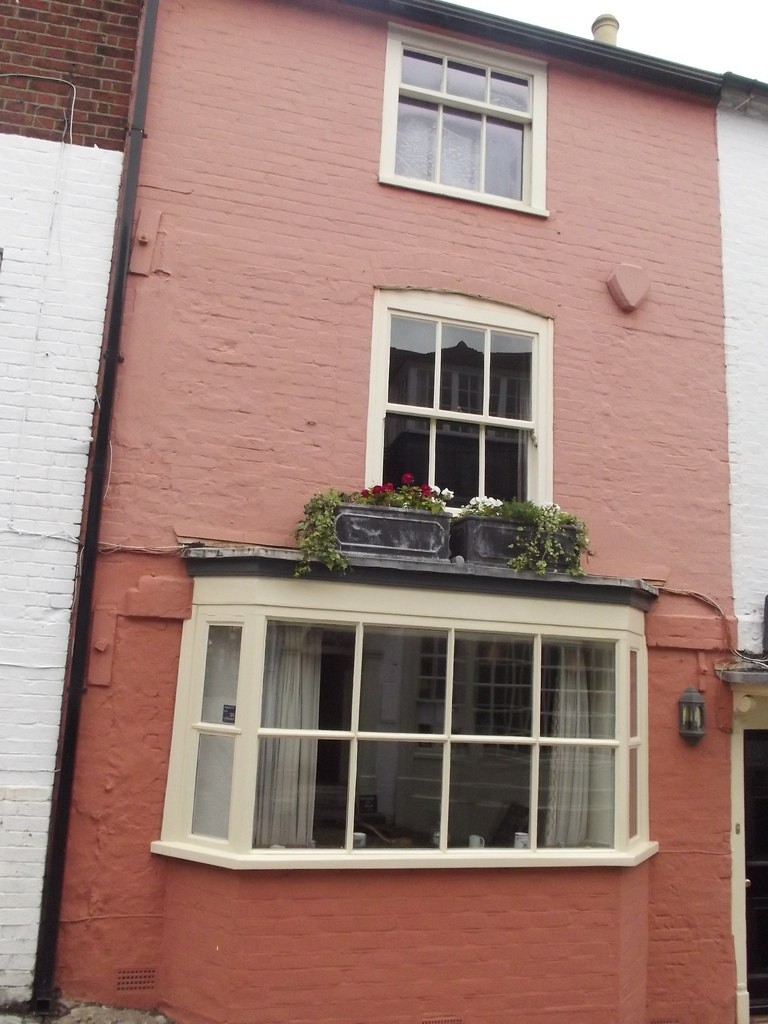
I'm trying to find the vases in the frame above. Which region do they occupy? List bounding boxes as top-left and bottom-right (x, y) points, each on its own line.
(449, 515), (584, 576)
(332, 501), (454, 563)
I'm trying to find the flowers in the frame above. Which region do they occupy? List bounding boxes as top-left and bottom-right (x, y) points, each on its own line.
(454, 495), (598, 577)
(294, 470), (455, 579)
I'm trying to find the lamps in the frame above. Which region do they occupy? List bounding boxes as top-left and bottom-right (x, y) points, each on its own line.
(677, 686), (709, 747)
